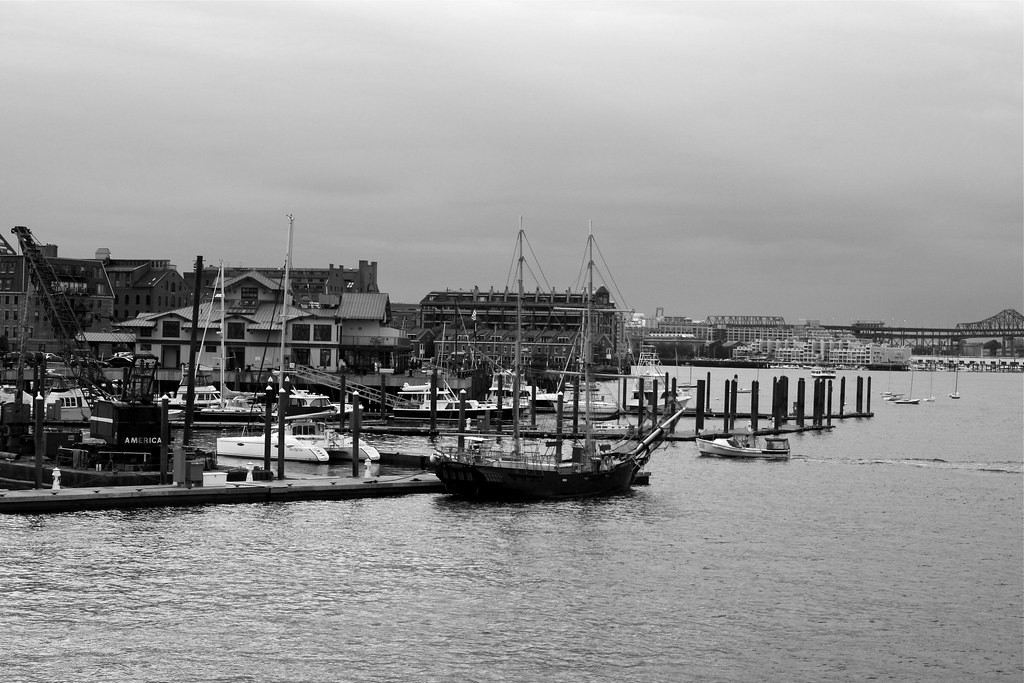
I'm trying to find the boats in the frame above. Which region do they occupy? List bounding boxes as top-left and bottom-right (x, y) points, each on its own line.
(695, 428), (792, 462)
(810, 368), (837, 379)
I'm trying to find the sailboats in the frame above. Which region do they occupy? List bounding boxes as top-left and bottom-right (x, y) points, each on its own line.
(423, 210), (690, 499)
(893, 365), (922, 405)
(948, 355), (961, 400)
(924, 364), (936, 402)
(0, 209), (688, 470)
(880, 365), (906, 401)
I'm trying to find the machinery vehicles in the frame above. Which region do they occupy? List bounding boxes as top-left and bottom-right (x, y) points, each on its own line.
(8, 223), (176, 466)
